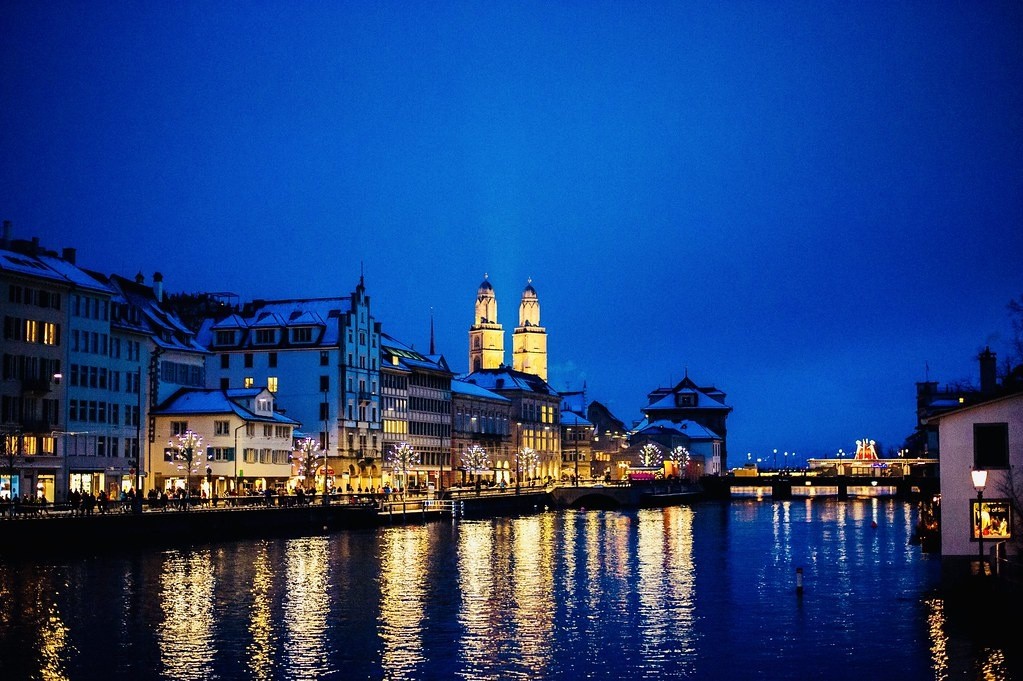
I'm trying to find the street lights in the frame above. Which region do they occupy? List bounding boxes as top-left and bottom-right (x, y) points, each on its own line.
(784, 452), (787, 468)
(234, 423), (254, 507)
(905, 447), (908, 474)
(837, 449), (845, 476)
(472, 412), (481, 487)
(773, 448), (777, 470)
(516, 419), (521, 494)
(971, 466), (988, 572)
(567, 419), (578, 486)
